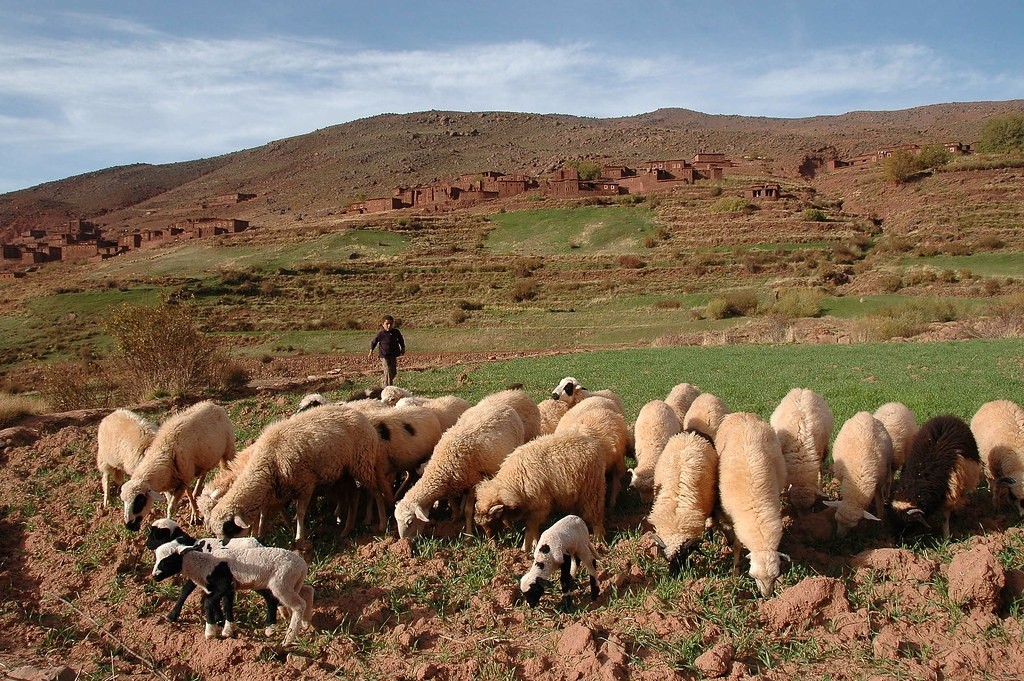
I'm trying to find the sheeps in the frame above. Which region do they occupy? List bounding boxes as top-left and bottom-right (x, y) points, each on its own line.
(627, 382), (784, 599)
(147, 518), (314, 647)
(96, 398), (236, 533)
(196, 386), (473, 546)
(395, 377), (635, 553)
(823, 402), (982, 544)
(970, 398), (1024, 518)
(770, 387), (833, 518)
(512, 515), (602, 610)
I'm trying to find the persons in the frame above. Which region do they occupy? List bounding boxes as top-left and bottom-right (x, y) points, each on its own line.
(367, 315), (405, 387)
